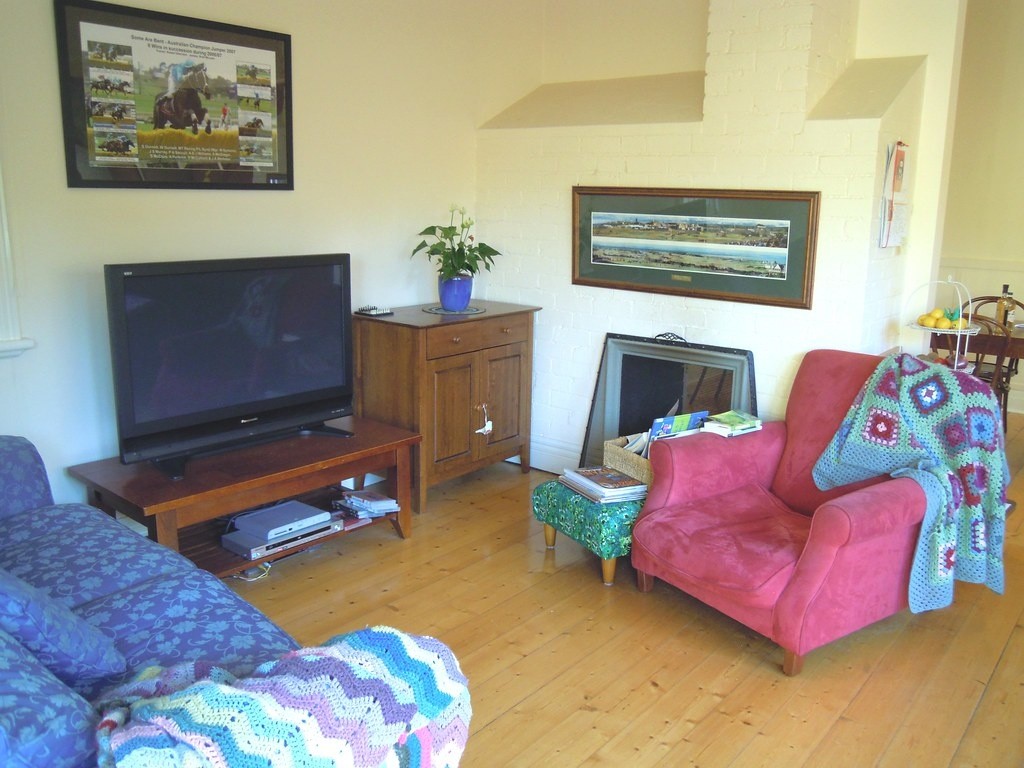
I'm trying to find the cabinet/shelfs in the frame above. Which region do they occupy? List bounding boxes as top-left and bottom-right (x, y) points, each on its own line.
(897, 276), (981, 374)
(352, 300), (541, 515)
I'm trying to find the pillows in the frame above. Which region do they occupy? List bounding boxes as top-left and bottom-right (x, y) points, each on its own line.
(0, 566), (126, 679)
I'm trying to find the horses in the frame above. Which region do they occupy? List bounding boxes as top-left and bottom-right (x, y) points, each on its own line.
(91, 79), (111, 93)
(153, 67), (212, 135)
(109, 80), (130, 96)
(99, 140), (135, 153)
(246, 120), (263, 127)
(247, 146), (264, 155)
(112, 109), (126, 121)
(95, 107), (106, 116)
(254, 98), (260, 110)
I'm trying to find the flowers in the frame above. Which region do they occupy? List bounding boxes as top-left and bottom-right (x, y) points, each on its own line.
(408, 206), (500, 283)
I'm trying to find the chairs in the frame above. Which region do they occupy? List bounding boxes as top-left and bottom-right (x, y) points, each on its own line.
(952, 297), (1024, 436)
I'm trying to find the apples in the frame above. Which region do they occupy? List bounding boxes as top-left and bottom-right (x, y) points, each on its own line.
(935, 357), (949, 365)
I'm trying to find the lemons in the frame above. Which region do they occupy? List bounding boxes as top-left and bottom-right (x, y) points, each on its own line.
(918, 309), (968, 329)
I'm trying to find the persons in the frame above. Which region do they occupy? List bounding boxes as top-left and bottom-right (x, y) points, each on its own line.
(219, 103), (231, 129)
(165, 59), (195, 97)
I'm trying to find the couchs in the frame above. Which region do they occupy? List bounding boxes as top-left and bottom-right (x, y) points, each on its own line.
(631, 348), (1012, 677)
(0, 435), (473, 768)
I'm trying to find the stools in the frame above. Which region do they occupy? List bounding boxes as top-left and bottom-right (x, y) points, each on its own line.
(532, 480), (645, 586)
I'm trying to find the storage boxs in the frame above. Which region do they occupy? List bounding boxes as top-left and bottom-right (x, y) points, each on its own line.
(604, 432), (652, 484)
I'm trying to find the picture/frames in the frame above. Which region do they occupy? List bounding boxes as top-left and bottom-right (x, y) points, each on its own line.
(572, 185), (822, 310)
(53, 1), (296, 191)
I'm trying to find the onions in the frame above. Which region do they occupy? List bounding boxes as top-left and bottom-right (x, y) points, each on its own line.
(947, 353), (969, 368)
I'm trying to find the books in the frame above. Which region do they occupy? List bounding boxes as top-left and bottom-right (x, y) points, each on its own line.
(622, 409), (762, 459)
(330, 490), (401, 531)
(558, 465), (649, 504)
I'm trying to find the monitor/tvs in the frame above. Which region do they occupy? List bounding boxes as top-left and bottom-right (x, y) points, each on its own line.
(104, 253), (356, 480)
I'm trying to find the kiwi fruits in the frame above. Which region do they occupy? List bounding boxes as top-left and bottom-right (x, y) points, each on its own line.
(917, 352), (938, 363)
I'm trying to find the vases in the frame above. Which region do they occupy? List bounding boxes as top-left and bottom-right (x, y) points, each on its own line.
(439, 274), (474, 315)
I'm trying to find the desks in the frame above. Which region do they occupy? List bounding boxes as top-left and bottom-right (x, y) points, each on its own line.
(64, 413), (423, 579)
(931, 324), (1024, 360)
